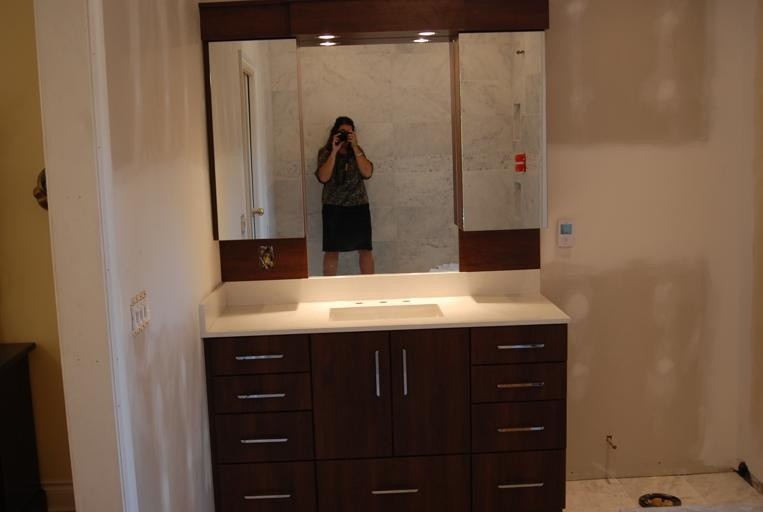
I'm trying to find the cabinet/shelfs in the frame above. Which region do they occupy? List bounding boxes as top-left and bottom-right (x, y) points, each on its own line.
(311, 328), (471, 511)
(472, 324), (567, 511)
(201, 333), (317, 511)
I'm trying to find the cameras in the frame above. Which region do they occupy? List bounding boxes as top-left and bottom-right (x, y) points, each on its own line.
(336, 130), (352, 141)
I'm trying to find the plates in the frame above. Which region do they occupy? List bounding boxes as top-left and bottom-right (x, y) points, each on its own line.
(638, 492), (682, 508)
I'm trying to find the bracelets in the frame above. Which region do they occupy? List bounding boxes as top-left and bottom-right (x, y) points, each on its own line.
(355, 152), (363, 157)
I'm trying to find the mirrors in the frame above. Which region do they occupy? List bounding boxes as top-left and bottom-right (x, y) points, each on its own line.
(299, 31), (460, 275)
(454, 31), (547, 231)
(208, 39), (307, 241)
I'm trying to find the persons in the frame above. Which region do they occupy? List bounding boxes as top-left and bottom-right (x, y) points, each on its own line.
(314, 116), (376, 275)
